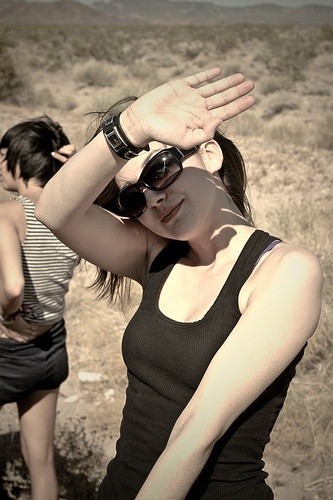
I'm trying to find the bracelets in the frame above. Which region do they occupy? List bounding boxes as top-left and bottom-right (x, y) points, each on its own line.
(102, 113), (150, 160)
(0, 303), (24, 324)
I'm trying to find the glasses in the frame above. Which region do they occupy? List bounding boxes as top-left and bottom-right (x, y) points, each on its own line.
(115, 145), (202, 220)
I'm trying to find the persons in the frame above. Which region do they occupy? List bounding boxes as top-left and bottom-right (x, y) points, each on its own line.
(0, 111), (82, 500)
(34, 66), (323, 500)
